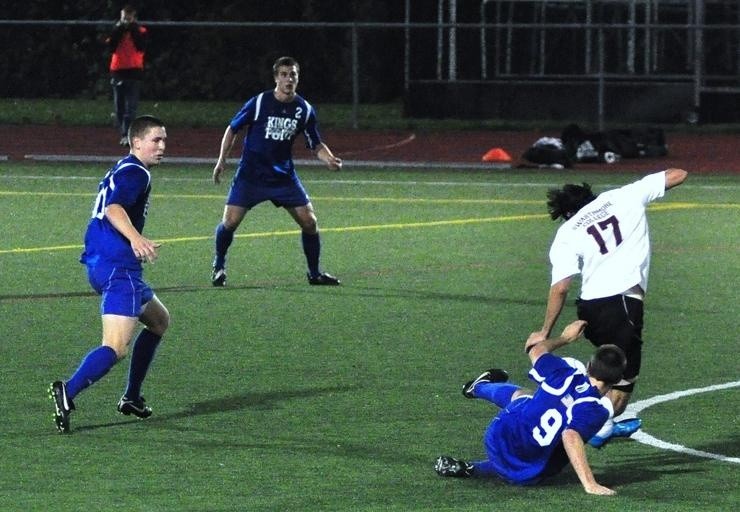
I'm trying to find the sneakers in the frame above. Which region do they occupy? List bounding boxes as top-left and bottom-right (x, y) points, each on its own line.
(590, 416), (643, 450)
(461, 368), (509, 398)
(116, 135), (129, 147)
(435, 455), (464, 476)
(210, 268), (228, 287)
(48, 381), (72, 434)
(115, 395), (153, 420)
(308, 272), (343, 288)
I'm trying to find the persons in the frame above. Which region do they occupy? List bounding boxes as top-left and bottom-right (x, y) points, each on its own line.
(207, 53), (346, 288)
(46, 113), (171, 438)
(523, 167), (690, 447)
(434, 318), (628, 497)
(102, 4), (147, 146)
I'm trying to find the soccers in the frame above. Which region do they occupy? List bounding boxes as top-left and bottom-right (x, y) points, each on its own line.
(562, 357), (587, 376)
